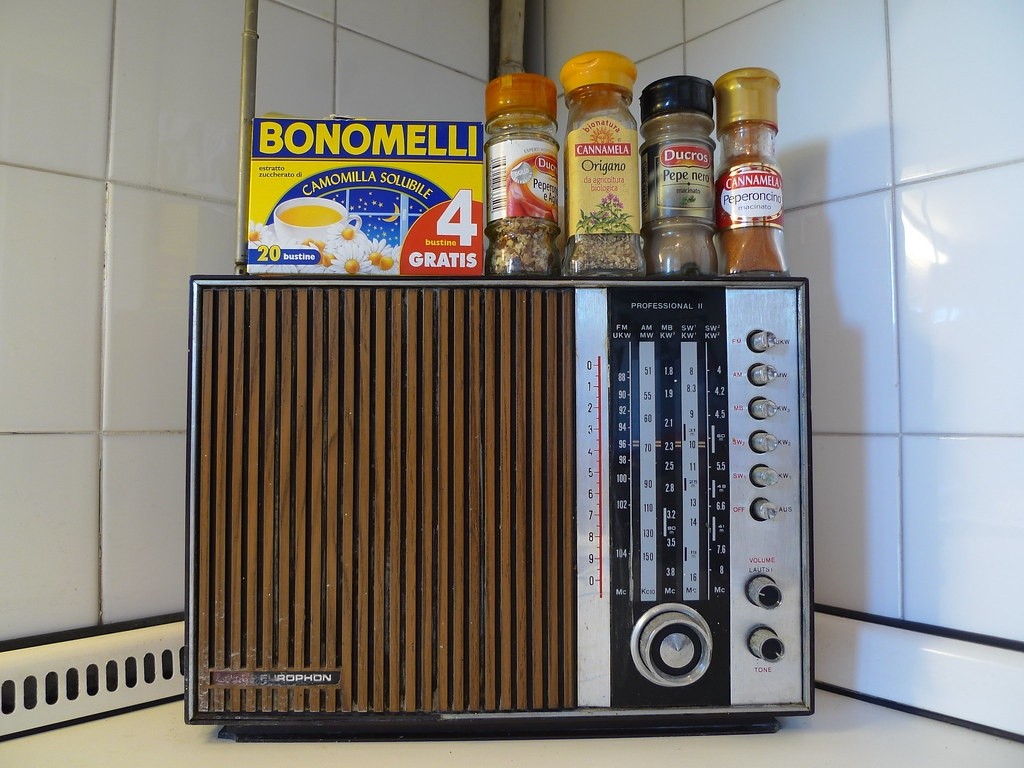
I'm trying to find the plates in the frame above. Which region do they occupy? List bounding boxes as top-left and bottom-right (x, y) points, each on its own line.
(261, 223), (371, 248)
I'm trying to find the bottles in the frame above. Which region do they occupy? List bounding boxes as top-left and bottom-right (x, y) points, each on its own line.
(715, 67), (791, 278)
(640, 73), (717, 280)
(485, 74), (561, 277)
(561, 51), (645, 277)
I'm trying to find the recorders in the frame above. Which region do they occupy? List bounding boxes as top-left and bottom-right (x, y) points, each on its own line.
(182, 276), (815, 744)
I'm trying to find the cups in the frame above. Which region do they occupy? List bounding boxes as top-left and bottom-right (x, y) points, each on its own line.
(275, 197), (363, 246)
